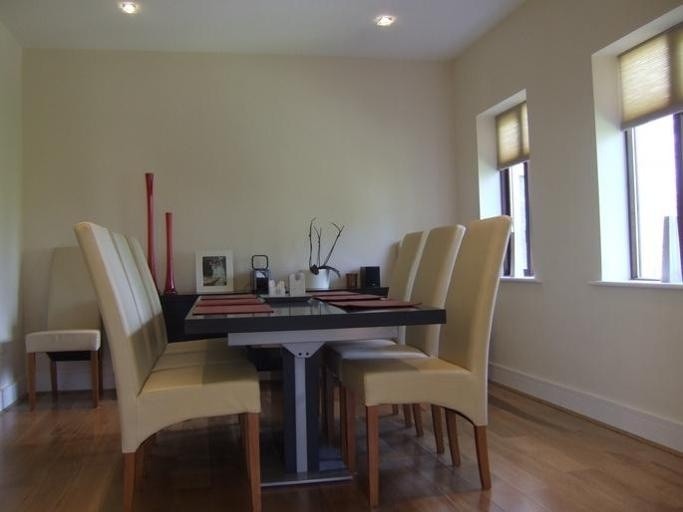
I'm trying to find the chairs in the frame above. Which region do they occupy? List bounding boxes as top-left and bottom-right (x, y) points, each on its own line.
(324, 224), (465, 469)
(316, 229), (426, 440)
(73, 220), (262, 511)
(343, 214), (511, 506)
(25, 246), (102, 411)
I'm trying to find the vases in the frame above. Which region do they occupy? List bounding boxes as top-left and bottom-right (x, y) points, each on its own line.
(144, 172), (157, 287)
(163, 212), (178, 295)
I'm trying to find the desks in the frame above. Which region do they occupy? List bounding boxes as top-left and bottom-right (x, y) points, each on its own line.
(158, 292), (389, 343)
(183, 290), (447, 488)
